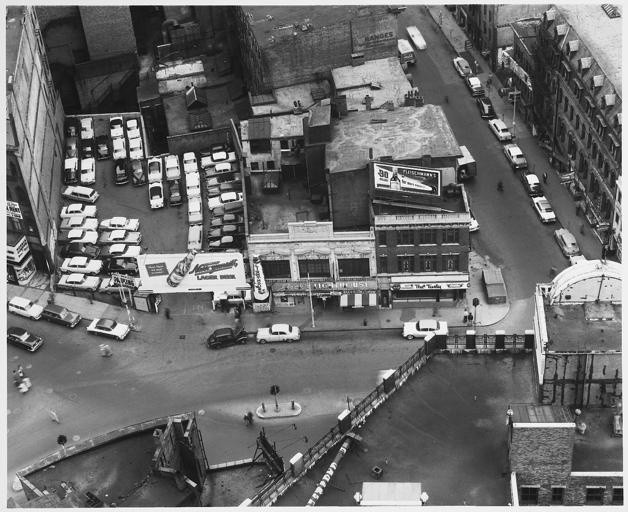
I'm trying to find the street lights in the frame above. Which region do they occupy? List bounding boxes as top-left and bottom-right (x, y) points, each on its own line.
(508, 85), (521, 139)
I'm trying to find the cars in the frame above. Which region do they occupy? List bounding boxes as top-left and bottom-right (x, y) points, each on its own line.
(521, 172), (542, 197)
(569, 254), (587, 266)
(584, 211), (596, 226)
(377, 369), (396, 385)
(401, 319), (448, 341)
(475, 95), (495, 119)
(503, 142), (526, 170)
(469, 208), (481, 232)
(554, 227), (579, 257)
(406, 24), (427, 51)
(465, 73), (484, 97)
(532, 195), (555, 223)
(453, 56), (473, 77)
(255, 323), (301, 344)
(488, 118), (511, 142)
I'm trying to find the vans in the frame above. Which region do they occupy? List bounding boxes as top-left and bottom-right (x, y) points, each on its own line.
(397, 37), (416, 65)
(454, 145), (477, 182)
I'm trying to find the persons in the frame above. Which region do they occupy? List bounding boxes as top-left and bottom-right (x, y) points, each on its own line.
(13, 370), (20, 383)
(468, 313), (472, 326)
(18, 365), (25, 378)
(464, 309), (469, 323)
(48, 408), (58, 423)
